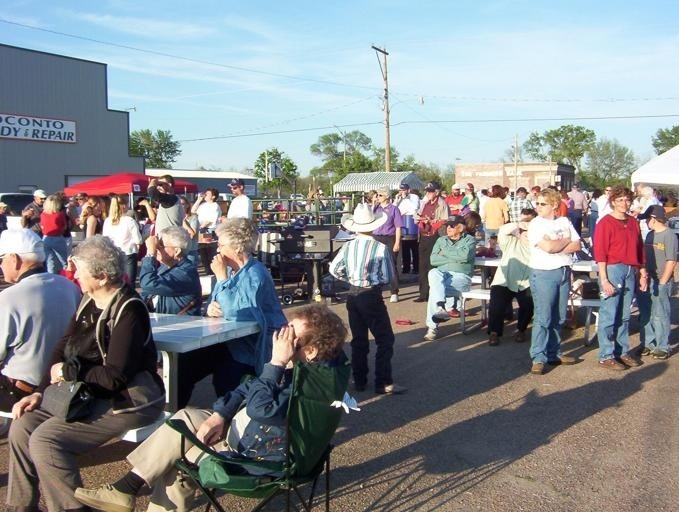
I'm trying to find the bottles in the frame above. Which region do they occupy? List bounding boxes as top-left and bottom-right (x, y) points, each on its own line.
(599, 283), (623, 300)
(490, 235), (497, 250)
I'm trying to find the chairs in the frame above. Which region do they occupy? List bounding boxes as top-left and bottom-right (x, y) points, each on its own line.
(164, 348), (352, 511)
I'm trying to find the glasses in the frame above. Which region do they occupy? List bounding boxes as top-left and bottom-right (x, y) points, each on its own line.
(230, 187), (237, 190)
(446, 224), (455, 228)
(536, 202), (549, 206)
(426, 189), (435, 192)
(614, 199), (631, 204)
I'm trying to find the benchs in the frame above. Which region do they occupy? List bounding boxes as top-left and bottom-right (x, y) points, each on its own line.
(1, 410), (173, 442)
(459, 289), (602, 349)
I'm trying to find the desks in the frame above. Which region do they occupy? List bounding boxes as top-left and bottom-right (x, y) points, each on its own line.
(473, 256), (598, 272)
(149, 312), (261, 422)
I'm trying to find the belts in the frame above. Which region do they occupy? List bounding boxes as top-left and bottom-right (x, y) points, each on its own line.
(6, 377), (35, 394)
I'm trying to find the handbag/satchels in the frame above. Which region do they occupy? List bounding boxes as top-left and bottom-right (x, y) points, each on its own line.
(579, 282), (600, 299)
(42, 381), (92, 422)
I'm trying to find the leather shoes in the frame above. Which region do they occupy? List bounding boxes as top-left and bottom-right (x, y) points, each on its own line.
(355, 385), (366, 391)
(413, 297), (428, 302)
(375, 384), (409, 393)
(446, 310), (460, 317)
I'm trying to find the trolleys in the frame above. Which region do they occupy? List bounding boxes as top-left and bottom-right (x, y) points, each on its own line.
(269, 235), (341, 307)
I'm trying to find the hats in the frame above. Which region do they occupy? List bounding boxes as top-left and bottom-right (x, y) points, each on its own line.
(0, 228), (44, 258)
(33, 190), (48, 198)
(400, 184), (409, 189)
(443, 215), (465, 224)
(425, 181), (440, 189)
(638, 205), (666, 219)
(227, 179), (244, 186)
(452, 184), (460, 189)
(341, 202), (388, 232)
(0, 202), (8, 207)
(74, 193), (88, 198)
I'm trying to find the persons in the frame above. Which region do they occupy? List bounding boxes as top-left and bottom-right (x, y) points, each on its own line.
(72, 302), (355, 512)
(1, 232), (166, 512)
(1, 226), (86, 420)
(1, 174), (679, 413)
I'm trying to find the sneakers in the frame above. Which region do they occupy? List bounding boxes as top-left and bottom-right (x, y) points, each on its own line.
(531, 363), (545, 373)
(653, 349), (670, 359)
(511, 329), (525, 341)
(635, 346), (650, 355)
(618, 355), (639, 366)
(548, 355), (576, 365)
(75, 483), (136, 512)
(432, 310), (450, 323)
(598, 358), (626, 370)
(460, 309), (470, 316)
(424, 327), (439, 341)
(489, 334), (498, 344)
(390, 294), (398, 303)
